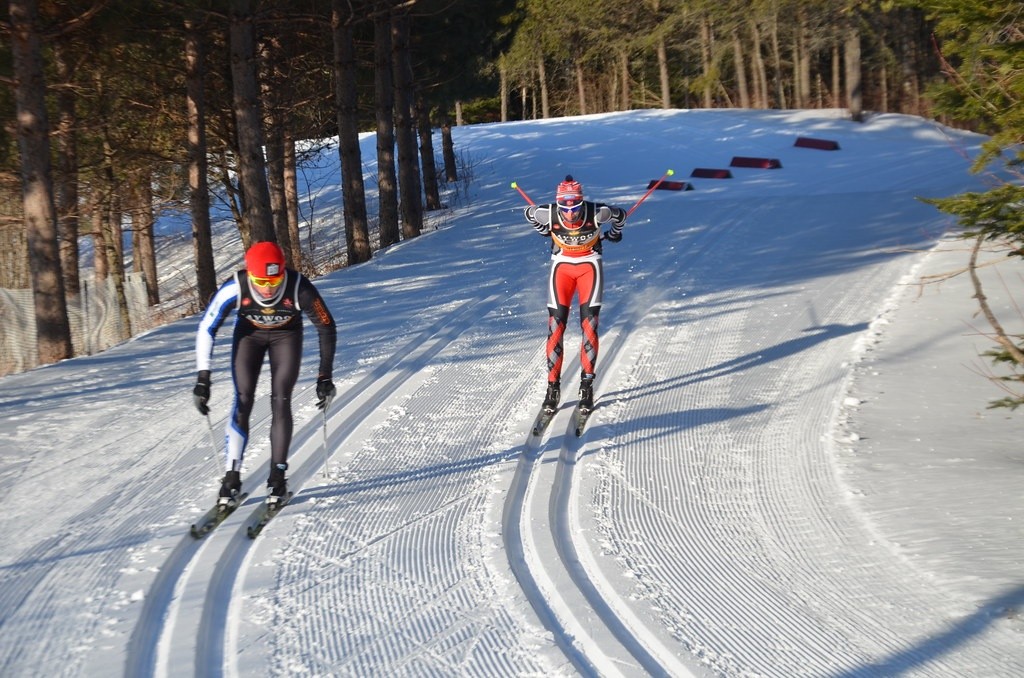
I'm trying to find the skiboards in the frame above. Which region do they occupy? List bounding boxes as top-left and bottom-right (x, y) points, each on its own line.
(532, 411), (590, 436)
(189, 490), (297, 539)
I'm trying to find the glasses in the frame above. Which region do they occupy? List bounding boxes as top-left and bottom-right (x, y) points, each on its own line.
(249, 272), (283, 287)
(557, 200), (583, 213)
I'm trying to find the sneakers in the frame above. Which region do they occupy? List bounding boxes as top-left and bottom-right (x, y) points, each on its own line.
(542, 388), (560, 412)
(219, 470), (242, 498)
(578, 384), (593, 412)
(267, 469), (287, 498)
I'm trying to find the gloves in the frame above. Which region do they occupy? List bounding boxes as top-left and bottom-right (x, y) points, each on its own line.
(604, 231), (622, 243)
(541, 231), (551, 237)
(315, 376), (336, 410)
(192, 378), (211, 415)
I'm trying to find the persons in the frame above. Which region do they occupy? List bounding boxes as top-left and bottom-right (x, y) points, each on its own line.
(523, 175), (626, 412)
(192, 241), (338, 507)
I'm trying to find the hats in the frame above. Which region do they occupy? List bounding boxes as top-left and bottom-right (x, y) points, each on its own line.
(556, 174), (584, 201)
(244, 241), (285, 278)
